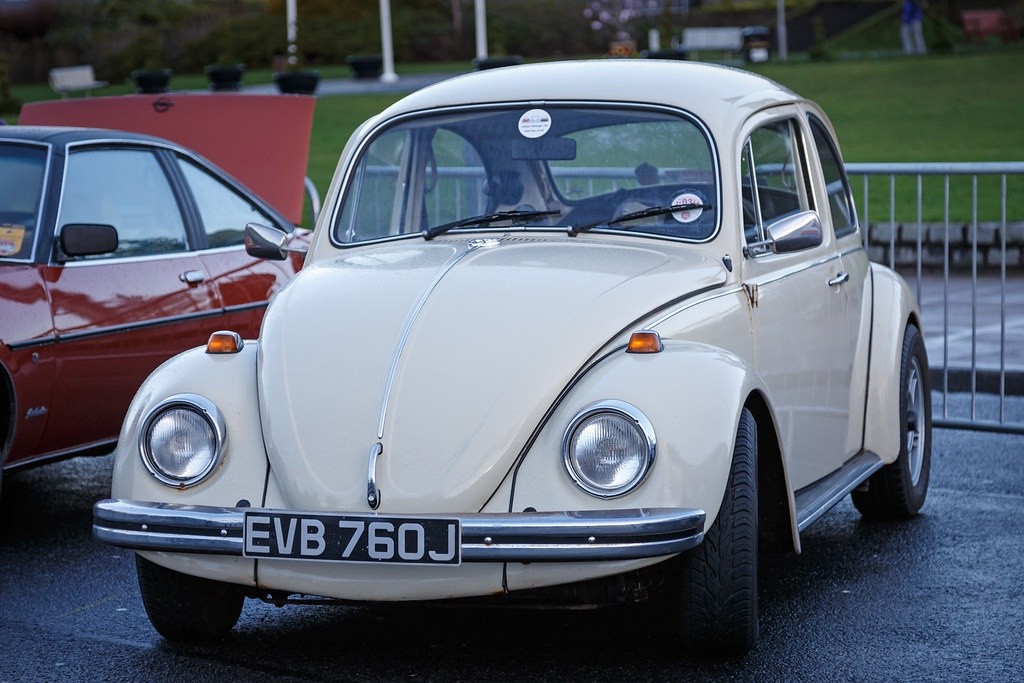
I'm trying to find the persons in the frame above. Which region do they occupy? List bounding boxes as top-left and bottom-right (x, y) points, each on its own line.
(899, 0), (929, 54)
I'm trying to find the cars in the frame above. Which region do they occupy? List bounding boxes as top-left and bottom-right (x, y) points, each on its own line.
(0, 92), (330, 480)
(92, 57), (932, 663)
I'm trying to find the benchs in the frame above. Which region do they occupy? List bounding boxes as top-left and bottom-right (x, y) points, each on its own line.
(547, 182), (798, 238)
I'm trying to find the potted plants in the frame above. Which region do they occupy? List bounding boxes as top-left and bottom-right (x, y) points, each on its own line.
(129, 37), (526, 95)
